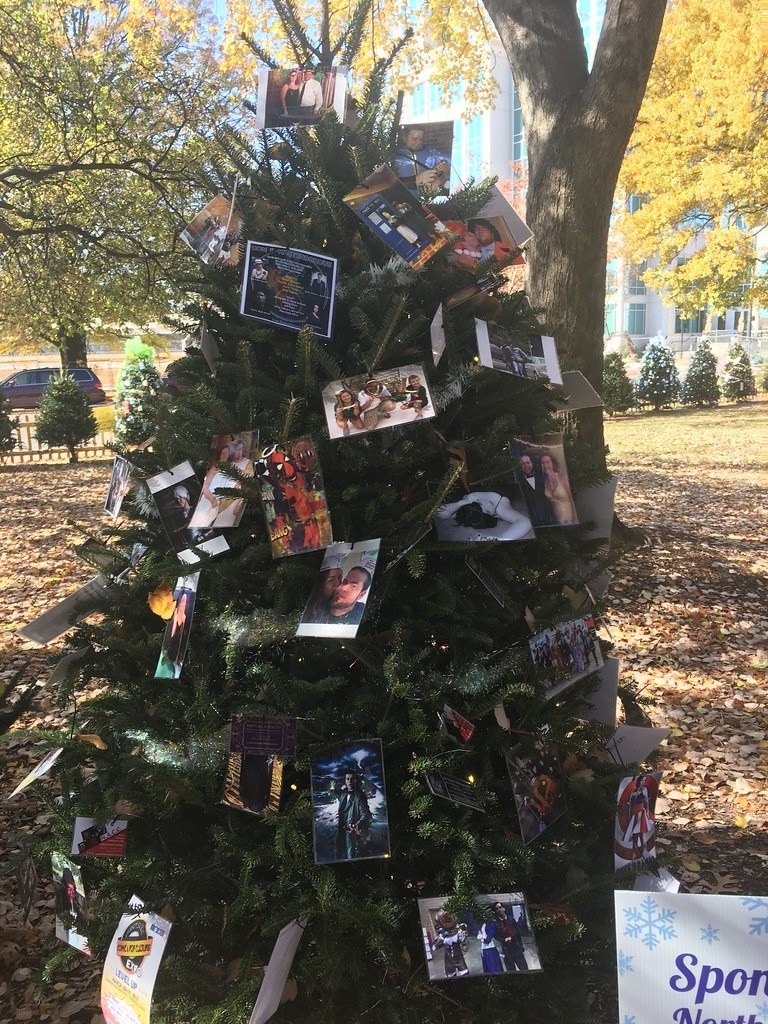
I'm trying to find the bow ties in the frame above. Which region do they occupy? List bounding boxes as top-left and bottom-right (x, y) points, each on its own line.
(523, 470), (535, 478)
(345, 791), (353, 794)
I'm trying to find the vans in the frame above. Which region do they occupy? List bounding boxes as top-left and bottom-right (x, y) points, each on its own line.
(0, 367), (107, 409)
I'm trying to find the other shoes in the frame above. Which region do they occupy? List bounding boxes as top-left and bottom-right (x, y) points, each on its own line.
(381, 411), (391, 418)
(343, 425), (349, 436)
(414, 414), (423, 421)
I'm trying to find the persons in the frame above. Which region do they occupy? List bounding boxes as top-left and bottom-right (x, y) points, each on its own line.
(401, 375), (428, 420)
(518, 449), (574, 529)
(445, 217), (525, 268)
(311, 273), (326, 323)
(503, 339), (536, 378)
(336, 772), (370, 860)
(334, 379), (396, 436)
(300, 567), (372, 624)
(431, 902), (529, 976)
(56, 868), (86, 927)
(248, 258), (270, 307)
(534, 624), (599, 689)
(192, 215), (225, 260)
(280, 67), (323, 115)
(621, 776), (656, 860)
(380, 201), (444, 247)
(76, 824), (122, 852)
(172, 485), (214, 551)
(187, 440), (254, 527)
(437, 491), (531, 541)
(391, 124), (451, 198)
(164, 593), (190, 664)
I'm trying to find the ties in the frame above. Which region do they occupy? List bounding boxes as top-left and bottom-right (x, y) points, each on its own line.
(298, 82), (306, 105)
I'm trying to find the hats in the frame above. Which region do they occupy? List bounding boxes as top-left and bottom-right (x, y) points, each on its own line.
(468, 218), (502, 243)
(253, 258), (263, 265)
